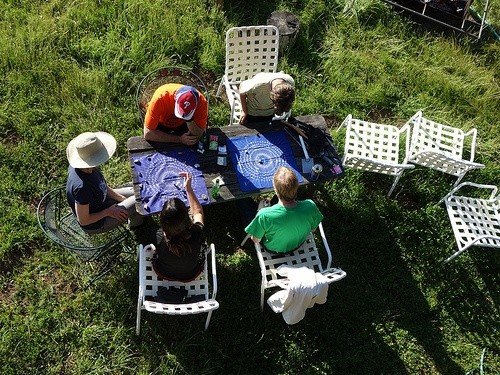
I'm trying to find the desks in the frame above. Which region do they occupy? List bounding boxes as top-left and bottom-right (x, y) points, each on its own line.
(127, 112), (347, 223)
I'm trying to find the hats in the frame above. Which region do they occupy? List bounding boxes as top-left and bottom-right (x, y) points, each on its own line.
(174, 86), (200, 121)
(67, 132), (117, 168)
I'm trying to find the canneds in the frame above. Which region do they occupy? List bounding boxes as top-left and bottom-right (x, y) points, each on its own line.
(309, 164), (323, 180)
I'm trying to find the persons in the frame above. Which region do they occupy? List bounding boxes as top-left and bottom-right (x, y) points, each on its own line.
(146, 171), (209, 282)
(144, 83), (208, 145)
(66, 132), (154, 233)
(239, 71), (296, 125)
(237, 166), (324, 254)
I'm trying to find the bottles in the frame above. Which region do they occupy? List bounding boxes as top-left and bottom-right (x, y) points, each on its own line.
(212, 179), (221, 199)
(199, 131), (207, 151)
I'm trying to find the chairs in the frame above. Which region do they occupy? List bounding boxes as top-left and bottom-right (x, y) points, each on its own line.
(396, 109), (489, 192)
(214, 24), (298, 125)
(238, 220), (348, 315)
(34, 184), (135, 291)
(133, 242), (219, 336)
(331, 110), (417, 197)
(135, 65), (211, 130)
(435, 179), (500, 266)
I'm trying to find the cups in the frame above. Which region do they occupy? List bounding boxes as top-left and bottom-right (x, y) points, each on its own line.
(311, 164), (323, 182)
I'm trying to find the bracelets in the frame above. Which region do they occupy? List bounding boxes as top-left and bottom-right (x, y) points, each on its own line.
(186, 117), (194, 123)
